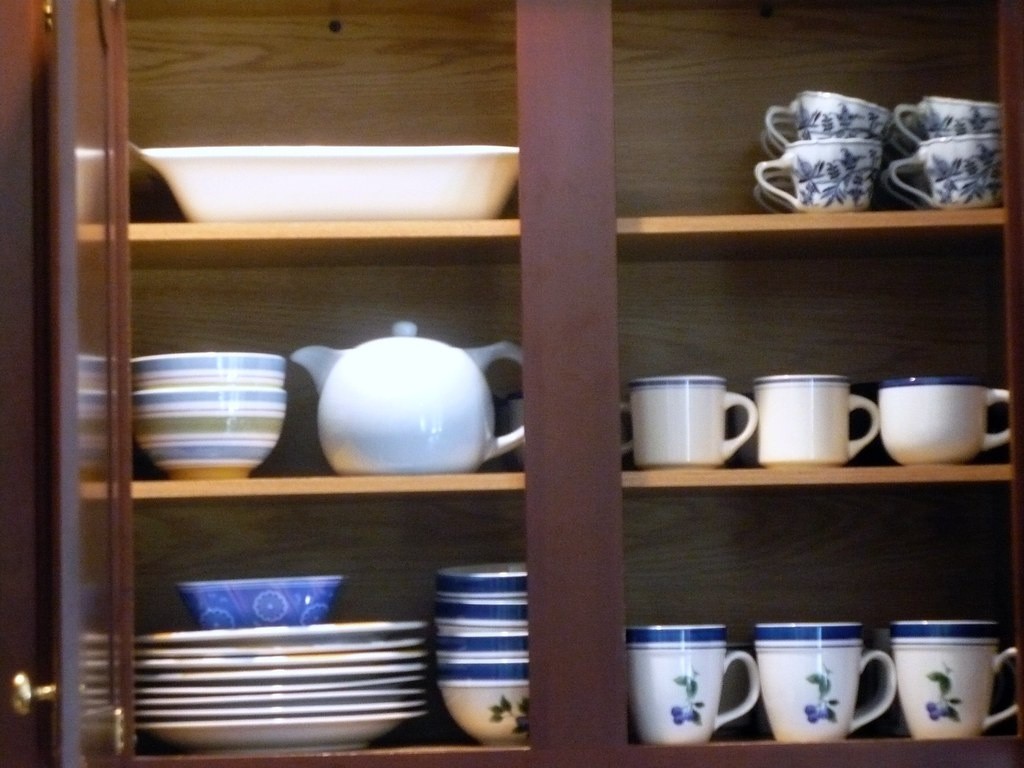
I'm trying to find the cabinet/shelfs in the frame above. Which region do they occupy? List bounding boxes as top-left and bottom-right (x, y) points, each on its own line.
(0, 1), (1024, 768)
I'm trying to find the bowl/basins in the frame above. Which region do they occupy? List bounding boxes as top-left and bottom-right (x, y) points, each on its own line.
(176, 574), (346, 630)
(433, 562), (528, 747)
(131, 351), (288, 387)
(133, 389), (288, 478)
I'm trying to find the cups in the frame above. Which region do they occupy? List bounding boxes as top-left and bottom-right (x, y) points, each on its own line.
(626, 624), (762, 744)
(894, 96), (1000, 157)
(890, 622), (1018, 739)
(754, 621), (899, 741)
(883, 134), (1000, 208)
(752, 372), (879, 465)
(754, 139), (883, 212)
(758, 90), (893, 161)
(878, 376), (1014, 464)
(629, 375), (759, 465)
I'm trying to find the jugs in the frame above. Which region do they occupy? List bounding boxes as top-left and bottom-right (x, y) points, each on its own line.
(290, 321), (523, 471)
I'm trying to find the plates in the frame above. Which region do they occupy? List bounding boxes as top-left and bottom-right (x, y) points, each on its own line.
(138, 147), (519, 220)
(134, 620), (429, 751)
